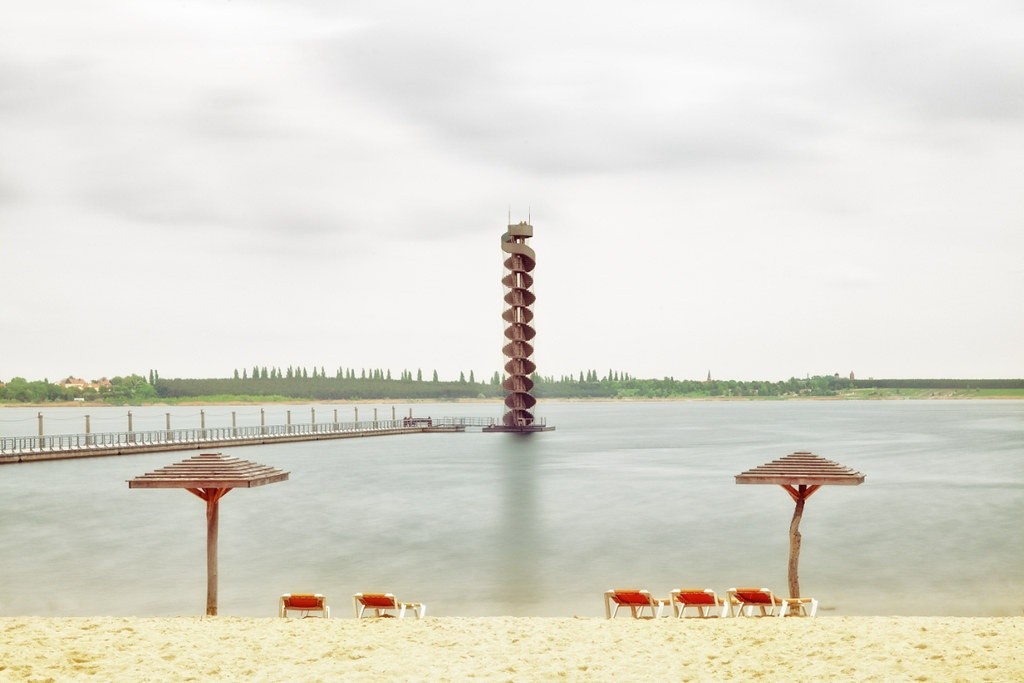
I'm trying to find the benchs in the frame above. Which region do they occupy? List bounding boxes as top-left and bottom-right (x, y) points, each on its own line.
(670, 588), (738, 617)
(353, 592), (426, 619)
(605, 586), (668, 617)
(727, 585), (818, 617)
(279, 593), (330, 619)
(404, 417), (434, 427)
